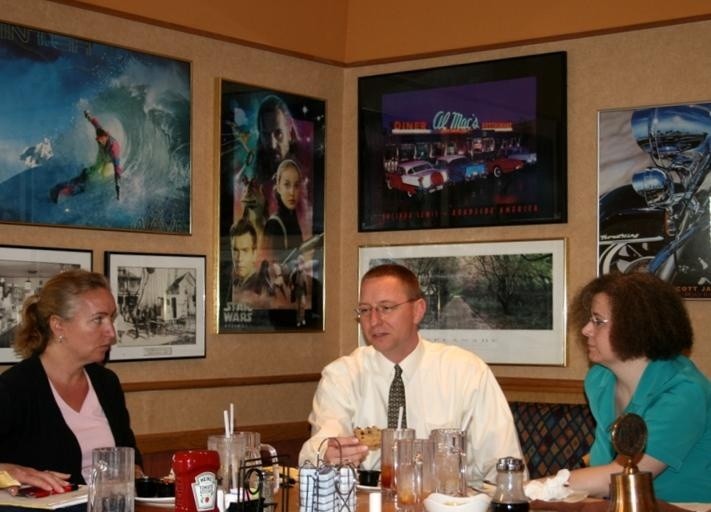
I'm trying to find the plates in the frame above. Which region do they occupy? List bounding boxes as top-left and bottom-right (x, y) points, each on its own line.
(356, 485), (380, 495)
(131, 494), (175, 509)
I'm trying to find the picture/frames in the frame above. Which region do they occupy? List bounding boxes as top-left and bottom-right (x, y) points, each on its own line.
(0, 244), (93, 366)
(104, 250), (206, 363)
(357, 237), (569, 368)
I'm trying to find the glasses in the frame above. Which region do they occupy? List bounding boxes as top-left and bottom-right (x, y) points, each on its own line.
(353, 300), (409, 315)
(589, 317), (612, 328)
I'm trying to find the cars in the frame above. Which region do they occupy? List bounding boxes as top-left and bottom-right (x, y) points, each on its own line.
(470, 151), (524, 178)
(496, 146), (537, 169)
(434, 155), (486, 184)
(384, 160), (449, 198)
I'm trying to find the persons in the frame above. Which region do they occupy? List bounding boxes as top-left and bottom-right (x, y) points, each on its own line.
(0, 268), (145, 496)
(231, 93), (308, 326)
(483, 271), (711, 511)
(297, 263), (531, 493)
(50, 110), (121, 205)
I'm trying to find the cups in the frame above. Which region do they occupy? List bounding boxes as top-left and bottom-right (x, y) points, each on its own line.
(205, 431), (279, 496)
(380, 426), (466, 511)
(89, 445), (136, 512)
(356, 469), (380, 487)
(171, 448), (218, 512)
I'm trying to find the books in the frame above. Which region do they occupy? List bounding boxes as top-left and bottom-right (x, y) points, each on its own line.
(0, 485), (90, 510)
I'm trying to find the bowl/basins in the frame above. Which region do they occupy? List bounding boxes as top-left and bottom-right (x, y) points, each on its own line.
(422, 490), (491, 512)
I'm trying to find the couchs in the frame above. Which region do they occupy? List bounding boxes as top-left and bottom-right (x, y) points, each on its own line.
(508, 401), (596, 478)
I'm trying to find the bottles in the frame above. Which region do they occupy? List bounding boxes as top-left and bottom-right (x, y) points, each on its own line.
(255, 470), (275, 512)
(298, 465), (358, 511)
(491, 457), (530, 512)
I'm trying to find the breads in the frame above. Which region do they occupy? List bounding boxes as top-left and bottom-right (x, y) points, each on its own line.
(352, 425), (387, 450)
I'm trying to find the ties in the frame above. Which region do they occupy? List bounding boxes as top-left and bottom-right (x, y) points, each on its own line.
(387, 365), (407, 428)
